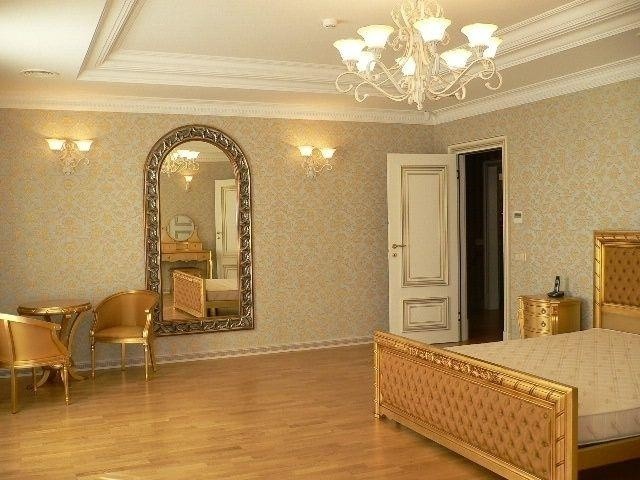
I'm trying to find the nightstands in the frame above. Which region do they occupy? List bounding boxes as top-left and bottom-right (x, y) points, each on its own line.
(517, 295), (581, 340)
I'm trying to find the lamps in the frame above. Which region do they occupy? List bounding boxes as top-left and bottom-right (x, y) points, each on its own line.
(160, 147), (200, 192)
(45, 138), (94, 176)
(299, 146), (337, 179)
(332, 0), (504, 112)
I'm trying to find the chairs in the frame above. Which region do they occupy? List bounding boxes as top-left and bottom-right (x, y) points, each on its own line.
(88, 290), (161, 377)
(0, 313), (72, 414)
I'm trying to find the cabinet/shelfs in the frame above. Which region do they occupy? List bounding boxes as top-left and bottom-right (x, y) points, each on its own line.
(160, 242), (210, 279)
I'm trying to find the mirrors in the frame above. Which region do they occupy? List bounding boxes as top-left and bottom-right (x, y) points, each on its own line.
(166, 214), (195, 242)
(143, 124), (254, 337)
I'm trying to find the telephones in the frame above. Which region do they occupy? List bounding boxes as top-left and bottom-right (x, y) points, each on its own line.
(547, 276), (564, 298)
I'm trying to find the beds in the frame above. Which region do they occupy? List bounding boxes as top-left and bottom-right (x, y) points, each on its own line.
(374, 232), (640, 479)
(173, 268), (241, 318)
